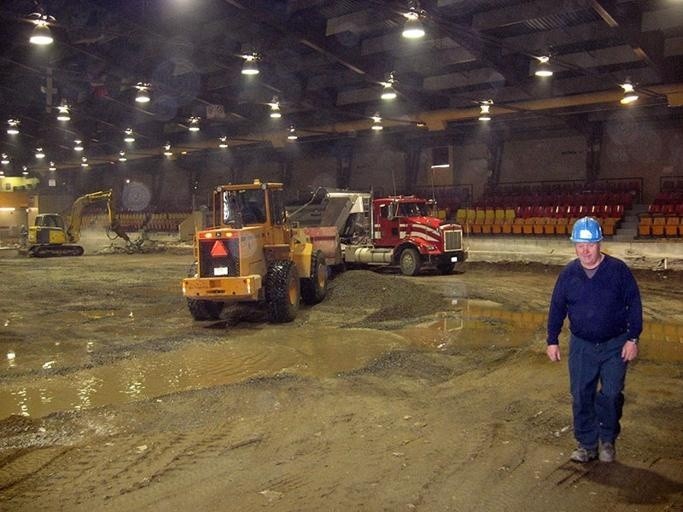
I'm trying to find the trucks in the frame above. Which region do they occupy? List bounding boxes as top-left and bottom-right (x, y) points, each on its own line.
(302, 183), (470, 275)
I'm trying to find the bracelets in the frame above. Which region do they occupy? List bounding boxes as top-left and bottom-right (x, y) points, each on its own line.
(627, 337), (639, 344)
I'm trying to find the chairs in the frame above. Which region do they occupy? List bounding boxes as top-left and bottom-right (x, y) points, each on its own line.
(428, 181), (683, 236)
(240, 205), (264, 224)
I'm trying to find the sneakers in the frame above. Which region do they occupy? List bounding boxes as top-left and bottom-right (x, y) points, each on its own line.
(570, 442), (616, 462)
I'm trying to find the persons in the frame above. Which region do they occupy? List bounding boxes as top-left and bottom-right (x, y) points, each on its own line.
(545, 217), (643, 463)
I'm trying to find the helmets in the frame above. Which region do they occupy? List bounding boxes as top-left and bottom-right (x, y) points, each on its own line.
(569, 217), (603, 242)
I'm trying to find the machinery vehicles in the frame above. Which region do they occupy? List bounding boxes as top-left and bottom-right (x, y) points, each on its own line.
(182, 178), (328, 324)
(20, 191), (130, 258)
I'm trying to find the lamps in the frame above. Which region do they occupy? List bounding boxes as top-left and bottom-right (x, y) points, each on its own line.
(1, 97), (173, 175)
(376, 70), (399, 100)
(477, 98), (495, 120)
(130, 80), (152, 103)
(401, 0), (426, 42)
(187, 94), (298, 149)
(240, 48), (264, 76)
(24, 1), (57, 45)
(534, 55), (639, 105)
